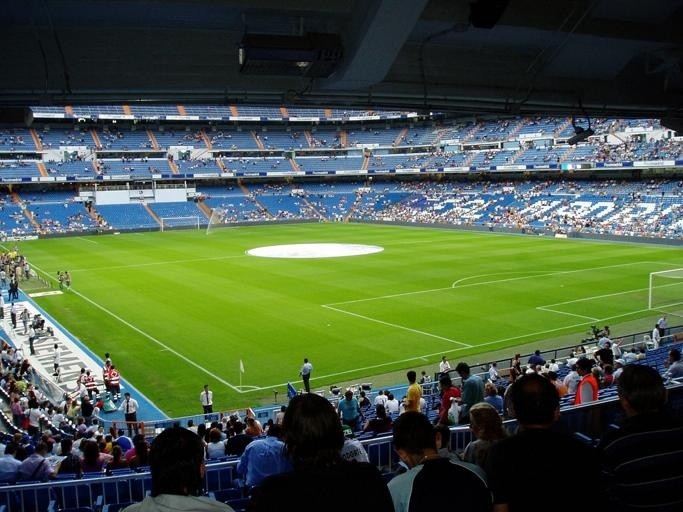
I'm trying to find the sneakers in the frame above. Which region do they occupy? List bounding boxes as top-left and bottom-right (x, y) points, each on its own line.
(126, 400), (129, 414)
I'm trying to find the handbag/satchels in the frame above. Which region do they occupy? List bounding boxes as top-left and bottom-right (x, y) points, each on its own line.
(24, 418), (29, 430)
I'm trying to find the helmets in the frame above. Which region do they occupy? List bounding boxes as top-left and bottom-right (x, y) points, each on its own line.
(118, 428), (124, 434)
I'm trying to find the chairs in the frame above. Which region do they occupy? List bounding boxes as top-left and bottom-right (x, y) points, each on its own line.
(0, 336), (683, 511)
(0, 113), (682, 245)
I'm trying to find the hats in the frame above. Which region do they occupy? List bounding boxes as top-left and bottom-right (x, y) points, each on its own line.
(22, 435), (29, 441)
(232, 422), (245, 431)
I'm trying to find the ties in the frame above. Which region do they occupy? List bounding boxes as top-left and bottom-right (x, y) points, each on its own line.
(206, 392), (209, 405)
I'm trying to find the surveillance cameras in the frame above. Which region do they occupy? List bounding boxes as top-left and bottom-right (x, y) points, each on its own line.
(566, 119), (594, 145)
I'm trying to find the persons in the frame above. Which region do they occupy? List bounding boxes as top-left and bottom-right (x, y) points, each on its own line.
(0, 118), (682, 239)
(0, 245), (119, 511)
(120, 313), (683, 512)
